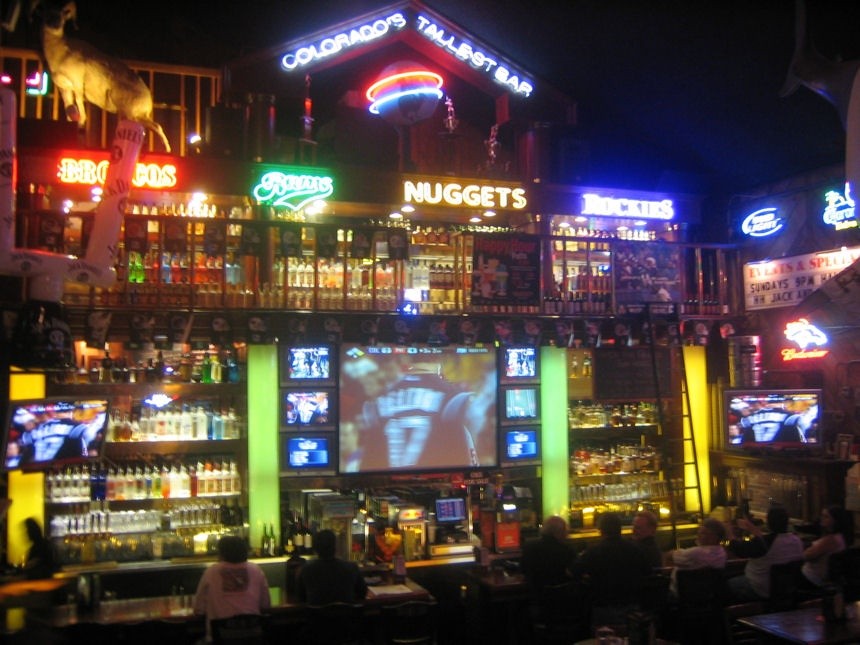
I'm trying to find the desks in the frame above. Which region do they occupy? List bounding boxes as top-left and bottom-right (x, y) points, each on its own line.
(23, 581), (442, 645)
(736, 601), (859, 645)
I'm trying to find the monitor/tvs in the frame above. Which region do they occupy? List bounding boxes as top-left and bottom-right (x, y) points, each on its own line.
(276, 341), (339, 477)
(722, 388), (823, 454)
(0, 396), (113, 471)
(497, 343), (542, 467)
(434, 496), (467, 531)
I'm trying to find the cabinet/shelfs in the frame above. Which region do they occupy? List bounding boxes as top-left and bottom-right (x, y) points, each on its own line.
(42, 382), (247, 547)
(569, 423), (665, 486)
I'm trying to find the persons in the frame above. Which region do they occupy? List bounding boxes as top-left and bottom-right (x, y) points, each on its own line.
(672, 505), (848, 608)
(295, 529), (374, 605)
(13, 403), (93, 460)
(516, 509), (663, 645)
(193, 536), (271, 645)
(286, 346), (538, 472)
(728, 393), (817, 444)
(24, 518), (60, 580)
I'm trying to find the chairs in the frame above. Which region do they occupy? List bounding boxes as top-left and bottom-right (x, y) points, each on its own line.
(210, 525), (860, 645)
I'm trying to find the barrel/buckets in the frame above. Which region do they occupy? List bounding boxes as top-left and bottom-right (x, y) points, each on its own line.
(726, 334), (762, 390)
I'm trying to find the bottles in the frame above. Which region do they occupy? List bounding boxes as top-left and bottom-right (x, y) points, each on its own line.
(569, 403), (679, 531)
(582, 352), (592, 378)
(48, 345), (243, 564)
(262, 521), (312, 556)
(570, 355), (580, 377)
(71, 254), (613, 315)
